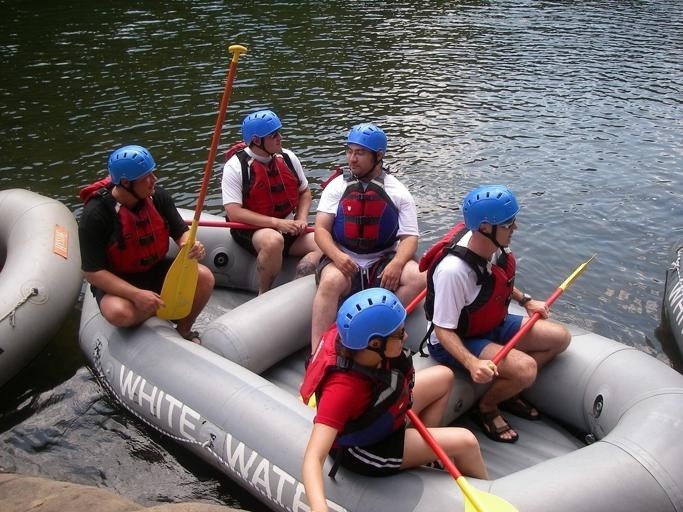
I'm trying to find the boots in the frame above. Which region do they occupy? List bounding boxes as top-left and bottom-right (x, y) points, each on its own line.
(471, 405), (518, 442)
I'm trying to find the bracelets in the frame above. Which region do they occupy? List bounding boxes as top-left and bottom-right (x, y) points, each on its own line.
(518, 293), (532, 306)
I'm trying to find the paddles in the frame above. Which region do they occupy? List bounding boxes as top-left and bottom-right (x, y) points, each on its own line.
(404, 407), (513, 512)
(486, 252), (598, 369)
(158, 44), (246, 322)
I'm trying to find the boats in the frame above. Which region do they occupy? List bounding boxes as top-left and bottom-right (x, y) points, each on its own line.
(77, 208), (682, 511)
(664, 237), (682, 357)
(0, 188), (83, 386)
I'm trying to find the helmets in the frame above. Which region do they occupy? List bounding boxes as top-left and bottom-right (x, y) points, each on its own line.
(107, 145), (157, 184)
(462, 185), (520, 230)
(346, 122), (387, 155)
(241, 110), (282, 143)
(336, 287), (407, 350)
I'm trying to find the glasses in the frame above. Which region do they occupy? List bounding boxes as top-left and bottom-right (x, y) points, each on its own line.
(500, 216), (517, 229)
(391, 328), (405, 340)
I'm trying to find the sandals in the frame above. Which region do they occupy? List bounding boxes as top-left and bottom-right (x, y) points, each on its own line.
(499, 395), (543, 420)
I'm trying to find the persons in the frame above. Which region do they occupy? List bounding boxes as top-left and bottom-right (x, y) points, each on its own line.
(79, 142), (215, 346)
(300, 287), (491, 512)
(305, 122), (429, 372)
(220, 110), (326, 298)
(424, 186), (571, 444)
